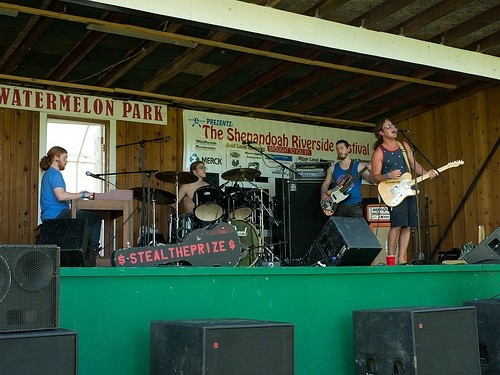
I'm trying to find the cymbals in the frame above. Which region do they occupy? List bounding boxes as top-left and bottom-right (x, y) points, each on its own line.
(221, 167), (261, 181)
(130, 187), (178, 205)
(154, 171), (199, 185)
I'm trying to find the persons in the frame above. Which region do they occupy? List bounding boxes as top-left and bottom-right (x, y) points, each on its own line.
(39, 146), (102, 259)
(321, 140), (375, 218)
(169, 161), (210, 213)
(372, 119), (437, 265)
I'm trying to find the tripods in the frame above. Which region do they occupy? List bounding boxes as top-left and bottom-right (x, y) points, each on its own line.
(399, 131), (447, 265)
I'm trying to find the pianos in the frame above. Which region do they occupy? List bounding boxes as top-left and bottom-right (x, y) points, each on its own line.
(67, 188), (135, 265)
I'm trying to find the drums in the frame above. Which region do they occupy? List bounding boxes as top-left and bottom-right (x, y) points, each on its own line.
(228, 189), (257, 220)
(193, 185), (227, 223)
(167, 211), (203, 245)
(229, 217), (261, 268)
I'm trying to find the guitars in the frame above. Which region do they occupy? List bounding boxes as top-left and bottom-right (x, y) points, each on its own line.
(376, 160), (465, 209)
(320, 166), (366, 216)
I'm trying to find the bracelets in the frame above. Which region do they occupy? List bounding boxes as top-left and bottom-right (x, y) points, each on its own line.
(81, 192), (85, 198)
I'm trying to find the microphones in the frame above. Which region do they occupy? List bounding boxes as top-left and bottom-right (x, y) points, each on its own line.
(86, 171), (106, 181)
(242, 141), (257, 144)
(394, 128), (410, 133)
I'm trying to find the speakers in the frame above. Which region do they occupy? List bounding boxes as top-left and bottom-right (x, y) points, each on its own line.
(353, 306), (481, 375)
(0, 327), (78, 375)
(303, 217), (382, 266)
(369, 223), (407, 266)
(0, 243), (60, 333)
(460, 227), (500, 264)
(150, 318), (294, 375)
(463, 299), (500, 375)
(284, 180), (335, 266)
(39, 218), (97, 267)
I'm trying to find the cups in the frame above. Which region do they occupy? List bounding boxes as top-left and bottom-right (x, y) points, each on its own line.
(386, 255), (395, 266)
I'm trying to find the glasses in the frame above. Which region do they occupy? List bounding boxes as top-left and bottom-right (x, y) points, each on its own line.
(380, 124), (395, 130)
(196, 166), (207, 170)
